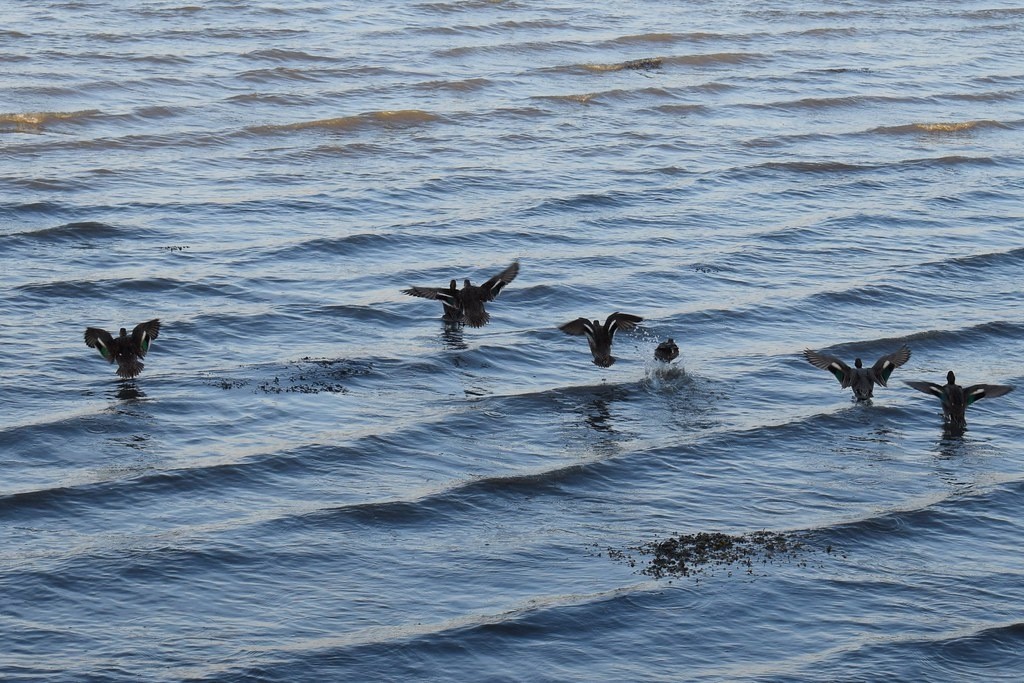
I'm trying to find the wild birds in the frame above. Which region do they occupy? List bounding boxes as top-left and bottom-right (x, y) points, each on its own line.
(803, 342), (913, 402)
(654, 337), (679, 363)
(556, 311), (644, 369)
(899, 370), (1017, 427)
(83, 318), (167, 381)
(398, 257), (521, 330)
